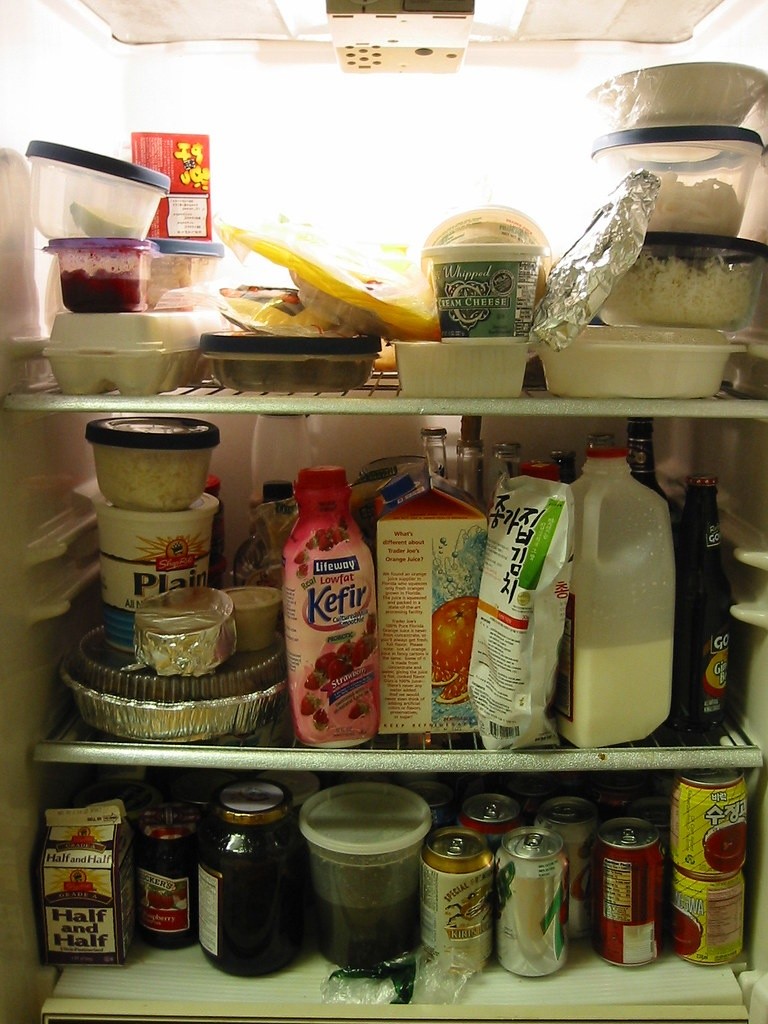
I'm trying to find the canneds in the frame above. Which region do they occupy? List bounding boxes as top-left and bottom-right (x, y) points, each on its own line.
(398, 768), (750, 977)
(63, 776), (314, 978)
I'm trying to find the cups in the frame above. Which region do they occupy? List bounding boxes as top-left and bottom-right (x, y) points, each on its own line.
(297, 781), (433, 972)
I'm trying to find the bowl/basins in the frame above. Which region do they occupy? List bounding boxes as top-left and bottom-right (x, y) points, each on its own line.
(422, 243), (544, 345)
(41, 311), (224, 392)
(591, 125), (764, 237)
(84, 417), (221, 514)
(94, 493), (224, 614)
(536, 324), (748, 398)
(388, 339), (533, 398)
(421, 204), (555, 312)
(146, 238), (226, 313)
(197, 329), (382, 393)
(587, 61), (768, 131)
(597, 232), (768, 335)
(24, 140), (172, 241)
(39, 237), (160, 314)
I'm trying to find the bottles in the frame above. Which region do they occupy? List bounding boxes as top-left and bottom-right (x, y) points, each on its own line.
(281, 464), (382, 749)
(233, 482), (294, 590)
(421, 417), (732, 746)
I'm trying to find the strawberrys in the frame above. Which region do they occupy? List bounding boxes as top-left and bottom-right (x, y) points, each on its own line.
(301, 613), (376, 730)
